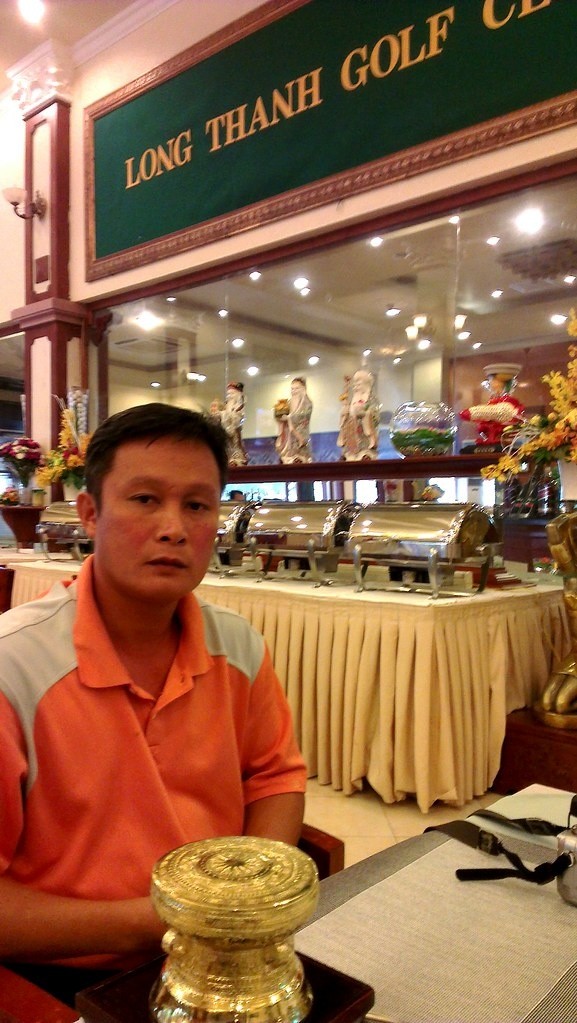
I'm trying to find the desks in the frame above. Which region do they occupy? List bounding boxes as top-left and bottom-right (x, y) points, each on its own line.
(73, 783), (577, 1023)
(10, 563), (572, 815)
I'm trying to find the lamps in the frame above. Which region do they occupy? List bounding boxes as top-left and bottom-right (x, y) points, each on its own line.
(3, 186), (48, 222)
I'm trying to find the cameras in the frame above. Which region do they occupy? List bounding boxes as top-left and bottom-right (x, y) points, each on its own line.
(555, 824), (577, 908)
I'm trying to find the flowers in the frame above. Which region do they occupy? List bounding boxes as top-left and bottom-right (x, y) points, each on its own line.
(1, 488), (18, 501)
(32, 389), (92, 485)
(481, 323), (577, 488)
(0, 435), (45, 486)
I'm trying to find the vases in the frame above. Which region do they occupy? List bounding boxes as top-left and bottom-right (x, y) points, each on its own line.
(19, 486), (33, 505)
(558, 458), (577, 513)
(4, 498), (17, 506)
(63, 474), (82, 501)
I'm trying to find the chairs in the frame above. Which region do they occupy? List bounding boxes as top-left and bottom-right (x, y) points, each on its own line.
(0, 822), (348, 1023)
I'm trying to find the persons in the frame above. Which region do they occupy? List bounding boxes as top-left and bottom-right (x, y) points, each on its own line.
(336, 370), (382, 462)
(460, 362), (523, 453)
(229, 490), (245, 502)
(212, 380), (251, 467)
(0, 402), (308, 998)
(274, 376), (315, 464)
